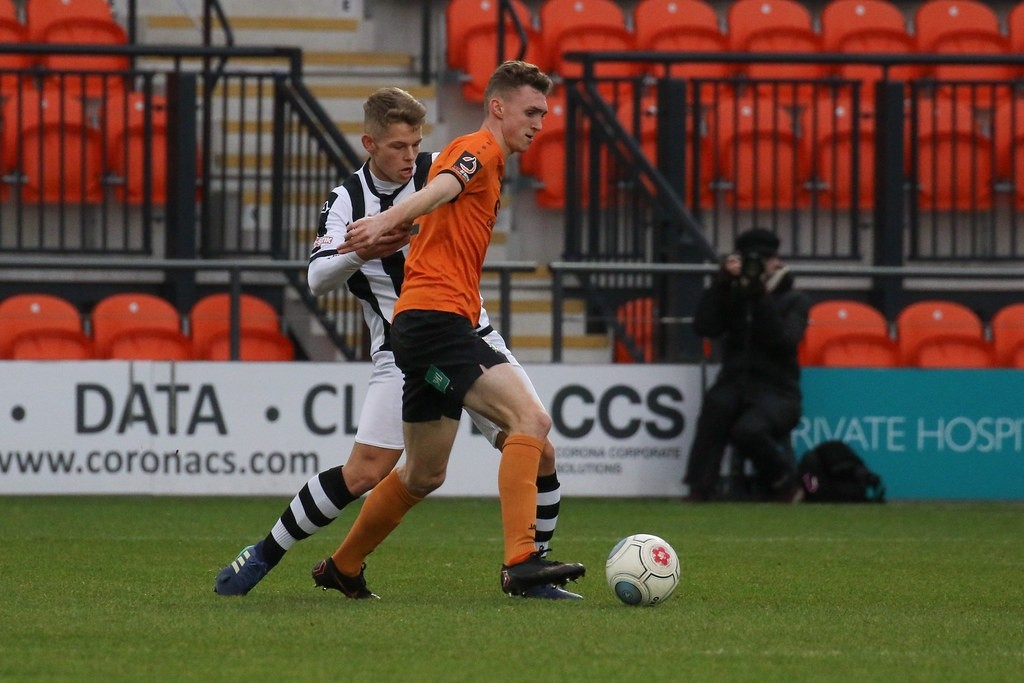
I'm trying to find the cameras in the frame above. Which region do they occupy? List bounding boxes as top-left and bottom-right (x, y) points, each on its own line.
(737, 251), (767, 282)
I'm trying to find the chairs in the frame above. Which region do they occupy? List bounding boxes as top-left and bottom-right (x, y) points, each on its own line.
(446, 0), (1023, 367)
(0, 0), (297, 365)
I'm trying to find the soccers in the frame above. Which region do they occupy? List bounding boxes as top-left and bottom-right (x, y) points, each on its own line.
(604, 532), (682, 608)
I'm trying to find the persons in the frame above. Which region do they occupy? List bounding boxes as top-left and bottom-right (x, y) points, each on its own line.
(683, 228), (804, 505)
(213, 88), (583, 599)
(312, 60), (586, 601)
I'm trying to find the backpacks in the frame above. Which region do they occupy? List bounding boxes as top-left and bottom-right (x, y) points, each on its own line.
(800, 440), (885, 505)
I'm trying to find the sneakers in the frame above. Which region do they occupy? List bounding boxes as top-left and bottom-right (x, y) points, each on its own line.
(522, 585), (581, 599)
(312, 556), (374, 599)
(216, 545), (273, 595)
(500, 544), (585, 595)
